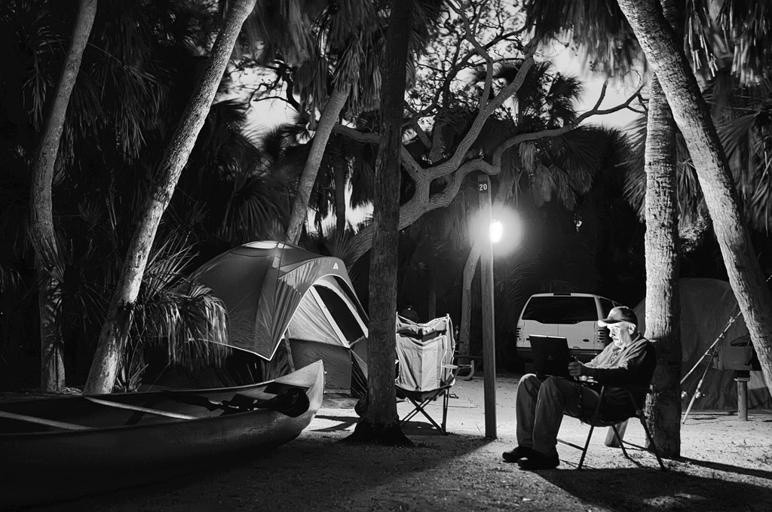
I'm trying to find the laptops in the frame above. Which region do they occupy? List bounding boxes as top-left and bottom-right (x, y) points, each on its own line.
(528, 334), (599, 385)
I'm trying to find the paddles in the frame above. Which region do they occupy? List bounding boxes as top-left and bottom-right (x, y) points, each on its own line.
(242, 385), (310, 419)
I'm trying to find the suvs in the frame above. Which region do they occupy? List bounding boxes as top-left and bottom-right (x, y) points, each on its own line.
(515, 293), (622, 374)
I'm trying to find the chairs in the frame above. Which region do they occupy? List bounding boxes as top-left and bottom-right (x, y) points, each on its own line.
(394, 310), (460, 437)
(555, 340), (667, 474)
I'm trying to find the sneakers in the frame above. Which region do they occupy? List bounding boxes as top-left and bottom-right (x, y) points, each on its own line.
(518, 451), (560, 468)
(502, 447), (532, 463)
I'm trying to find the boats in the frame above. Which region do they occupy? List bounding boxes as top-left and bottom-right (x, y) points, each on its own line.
(0, 359), (323, 509)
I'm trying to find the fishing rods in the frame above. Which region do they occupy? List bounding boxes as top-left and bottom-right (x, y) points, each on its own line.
(679, 273), (772, 384)
(680, 246), (767, 424)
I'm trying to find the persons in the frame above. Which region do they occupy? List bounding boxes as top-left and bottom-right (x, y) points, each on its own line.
(502, 305), (659, 468)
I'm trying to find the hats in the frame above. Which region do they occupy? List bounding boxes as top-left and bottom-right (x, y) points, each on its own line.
(601, 306), (638, 325)
